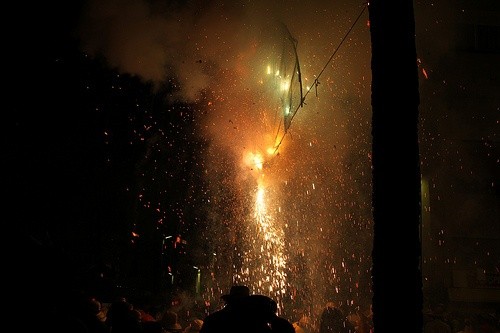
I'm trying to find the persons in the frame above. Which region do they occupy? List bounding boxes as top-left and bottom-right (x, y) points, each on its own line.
(3, 229), (467, 333)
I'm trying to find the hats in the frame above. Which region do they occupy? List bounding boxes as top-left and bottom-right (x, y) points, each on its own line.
(220, 285), (253, 307)
